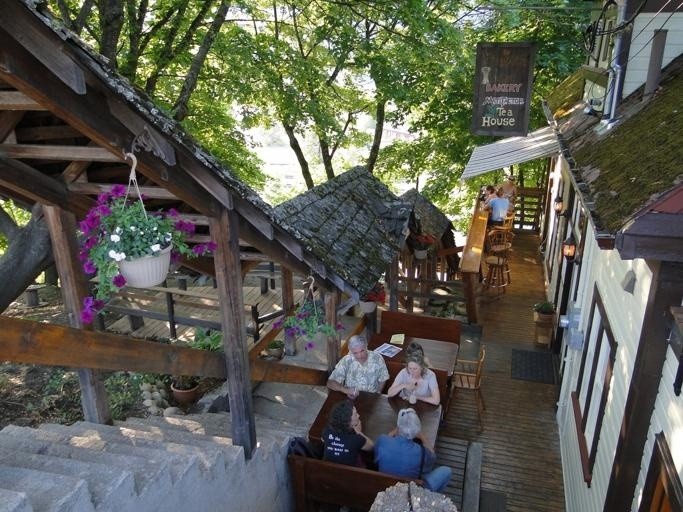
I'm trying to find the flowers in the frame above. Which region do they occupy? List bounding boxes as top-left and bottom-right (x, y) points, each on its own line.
(80, 185), (218, 324)
(364, 282), (385, 305)
(272, 299), (345, 351)
(414, 231), (435, 250)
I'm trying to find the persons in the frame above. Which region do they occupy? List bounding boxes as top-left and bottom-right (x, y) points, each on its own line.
(482, 175), (517, 226)
(407, 342), (432, 367)
(374, 408), (452, 494)
(388, 355), (440, 406)
(320, 401), (374, 468)
(327, 335), (390, 400)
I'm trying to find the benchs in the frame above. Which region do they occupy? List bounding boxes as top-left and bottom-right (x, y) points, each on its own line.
(287, 452), (425, 512)
(380, 310), (461, 347)
(382, 361), (447, 420)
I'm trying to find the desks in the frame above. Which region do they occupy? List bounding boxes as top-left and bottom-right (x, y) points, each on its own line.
(367, 335), (459, 377)
(308, 390), (443, 453)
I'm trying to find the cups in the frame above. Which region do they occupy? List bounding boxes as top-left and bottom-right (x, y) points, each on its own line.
(348, 386), (360, 399)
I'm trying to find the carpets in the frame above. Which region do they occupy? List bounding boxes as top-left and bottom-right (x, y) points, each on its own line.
(510, 348), (557, 385)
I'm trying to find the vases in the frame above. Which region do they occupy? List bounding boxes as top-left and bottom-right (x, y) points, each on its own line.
(358, 299), (377, 313)
(414, 248), (427, 260)
(118, 242), (174, 288)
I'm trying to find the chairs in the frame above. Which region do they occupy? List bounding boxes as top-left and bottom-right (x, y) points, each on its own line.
(481, 202), (516, 300)
(445, 350), (486, 431)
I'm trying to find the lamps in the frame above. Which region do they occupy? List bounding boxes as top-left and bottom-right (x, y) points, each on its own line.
(560, 236), (582, 265)
(554, 196), (569, 219)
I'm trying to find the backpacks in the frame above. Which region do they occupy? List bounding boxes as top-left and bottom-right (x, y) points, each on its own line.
(287, 434), (321, 462)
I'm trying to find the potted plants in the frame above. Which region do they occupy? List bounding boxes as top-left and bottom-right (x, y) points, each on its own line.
(535, 301), (554, 321)
(266, 340), (285, 358)
(170, 328), (222, 407)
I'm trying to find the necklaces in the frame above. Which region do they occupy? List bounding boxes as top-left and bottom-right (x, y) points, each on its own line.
(410, 380), (422, 386)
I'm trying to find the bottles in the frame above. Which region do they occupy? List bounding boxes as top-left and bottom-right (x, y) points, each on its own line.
(409, 388), (416, 404)
